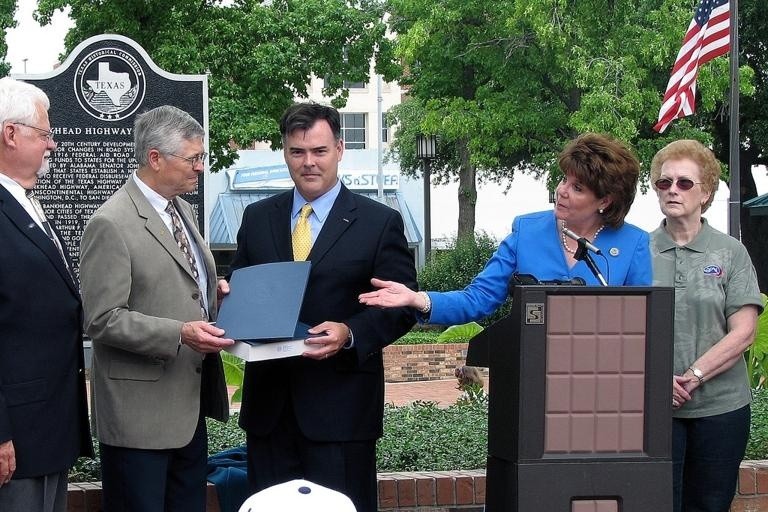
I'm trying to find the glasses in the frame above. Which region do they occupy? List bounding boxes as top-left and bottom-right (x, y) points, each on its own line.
(13, 122), (55, 142)
(169, 151), (208, 166)
(654, 179), (705, 190)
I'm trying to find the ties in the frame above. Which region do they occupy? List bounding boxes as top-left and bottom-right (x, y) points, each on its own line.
(25, 190), (75, 285)
(292, 204), (315, 262)
(165, 201), (209, 322)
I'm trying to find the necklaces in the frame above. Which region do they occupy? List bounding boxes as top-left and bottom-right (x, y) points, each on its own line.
(559, 220), (608, 254)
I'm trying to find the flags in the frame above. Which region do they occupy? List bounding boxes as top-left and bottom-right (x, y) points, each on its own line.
(651, 0), (733, 134)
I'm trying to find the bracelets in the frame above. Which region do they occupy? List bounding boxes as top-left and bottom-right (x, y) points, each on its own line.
(343, 328), (352, 347)
(416, 291), (432, 314)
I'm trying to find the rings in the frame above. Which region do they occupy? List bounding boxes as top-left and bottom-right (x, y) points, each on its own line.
(325, 353), (328, 359)
(672, 399), (677, 406)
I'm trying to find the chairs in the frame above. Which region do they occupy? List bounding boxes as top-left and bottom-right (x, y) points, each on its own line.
(238, 478), (358, 512)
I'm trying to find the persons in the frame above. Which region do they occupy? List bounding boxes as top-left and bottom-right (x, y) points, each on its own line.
(0, 75), (94, 512)
(646, 140), (764, 512)
(79, 106), (235, 512)
(227, 100), (420, 512)
(357, 134), (654, 327)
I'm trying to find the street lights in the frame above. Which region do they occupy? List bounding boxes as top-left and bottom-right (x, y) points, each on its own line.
(415, 127), (441, 270)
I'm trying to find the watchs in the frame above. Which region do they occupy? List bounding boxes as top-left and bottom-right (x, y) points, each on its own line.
(689, 366), (704, 386)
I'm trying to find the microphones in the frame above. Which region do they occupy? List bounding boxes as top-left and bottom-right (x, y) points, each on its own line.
(562, 228), (601, 255)
